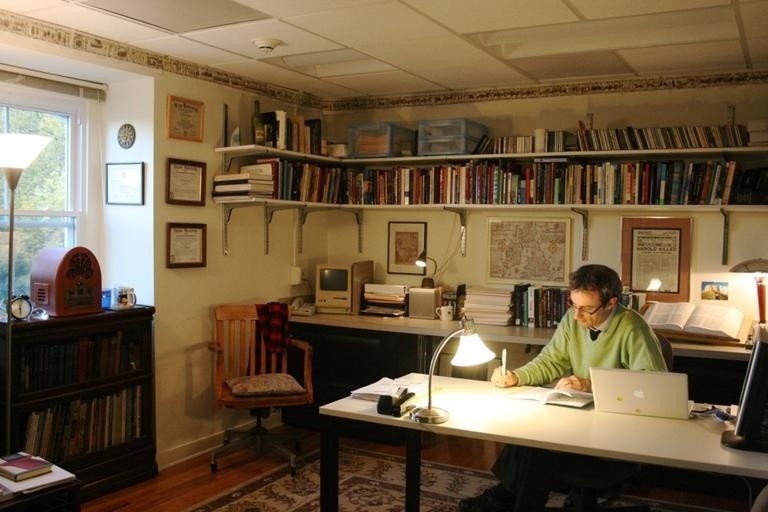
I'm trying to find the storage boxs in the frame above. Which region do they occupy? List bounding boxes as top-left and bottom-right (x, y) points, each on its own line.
(747, 120), (768, 145)
(419, 118), (488, 155)
(348, 122), (416, 157)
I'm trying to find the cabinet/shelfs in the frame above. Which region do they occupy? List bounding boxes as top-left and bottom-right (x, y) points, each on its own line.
(212, 146), (768, 210)
(0, 306), (156, 503)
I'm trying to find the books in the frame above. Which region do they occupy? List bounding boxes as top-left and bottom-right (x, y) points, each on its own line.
(642, 300), (744, 341)
(212, 110), (413, 206)
(458, 279), (646, 330)
(516, 384), (592, 410)
(11, 327), (144, 463)
(0, 455), (52, 484)
(413, 122), (766, 206)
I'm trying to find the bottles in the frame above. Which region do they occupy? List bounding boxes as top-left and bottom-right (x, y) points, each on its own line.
(249, 96), (266, 147)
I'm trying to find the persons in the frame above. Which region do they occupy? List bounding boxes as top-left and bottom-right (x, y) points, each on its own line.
(457, 262), (669, 510)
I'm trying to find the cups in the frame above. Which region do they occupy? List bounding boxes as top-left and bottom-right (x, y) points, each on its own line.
(532, 128), (545, 155)
(110, 285), (136, 310)
(434, 305), (454, 322)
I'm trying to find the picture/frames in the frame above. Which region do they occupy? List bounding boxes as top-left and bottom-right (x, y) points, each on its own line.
(166, 95), (204, 142)
(166, 223), (207, 268)
(388, 222), (427, 275)
(105, 162), (144, 205)
(621, 215), (693, 304)
(487, 217), (571, 287)
(165, 158), (206, 206)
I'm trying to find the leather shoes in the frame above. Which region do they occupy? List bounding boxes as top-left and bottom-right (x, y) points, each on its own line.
(459, 486), (514, 512)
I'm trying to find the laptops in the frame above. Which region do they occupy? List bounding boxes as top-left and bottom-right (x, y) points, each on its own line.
(590, 367), (694, 419)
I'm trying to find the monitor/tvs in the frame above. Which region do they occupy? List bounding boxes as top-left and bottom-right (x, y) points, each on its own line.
(721, 328), (768, 453)
(316, 264), (352, 314)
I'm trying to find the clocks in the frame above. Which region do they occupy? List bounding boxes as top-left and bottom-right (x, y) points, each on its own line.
(117, 124), (135, 149)
(11, 295), (31, 320)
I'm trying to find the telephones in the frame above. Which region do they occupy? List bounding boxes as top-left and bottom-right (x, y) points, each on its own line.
(292, 298), (315, 316)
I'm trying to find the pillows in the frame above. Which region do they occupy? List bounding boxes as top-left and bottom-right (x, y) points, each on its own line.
(226, 373), (306, 395)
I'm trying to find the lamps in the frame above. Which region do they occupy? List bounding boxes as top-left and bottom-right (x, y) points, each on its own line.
(0, 133), (51, 458)
(415, 252), (436, 287)
(411, 316), (496, 423)
(730, 258), (768, 324)
(251, 38), (285, 53)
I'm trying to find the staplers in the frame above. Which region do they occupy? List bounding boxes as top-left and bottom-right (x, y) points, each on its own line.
(393, 392), (417, 417)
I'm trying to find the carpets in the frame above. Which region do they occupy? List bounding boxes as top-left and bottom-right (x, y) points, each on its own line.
(181, 443), (566, 512)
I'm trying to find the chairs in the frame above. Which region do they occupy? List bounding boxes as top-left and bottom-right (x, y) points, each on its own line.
(546, 333), (673, 512)
(210, 305), (313, 474)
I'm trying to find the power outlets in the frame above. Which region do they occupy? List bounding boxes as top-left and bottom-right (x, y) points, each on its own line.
(290, 265), (306, 285)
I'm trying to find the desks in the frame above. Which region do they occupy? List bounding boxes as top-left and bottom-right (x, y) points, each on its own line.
(318, 372), (768, 512)
(290, 312), (753, 363)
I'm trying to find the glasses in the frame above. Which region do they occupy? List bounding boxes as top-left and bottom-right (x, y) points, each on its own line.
(566, 297), (603, 314)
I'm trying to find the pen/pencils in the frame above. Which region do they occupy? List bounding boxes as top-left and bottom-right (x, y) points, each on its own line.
(501, 348), (508, 389)
